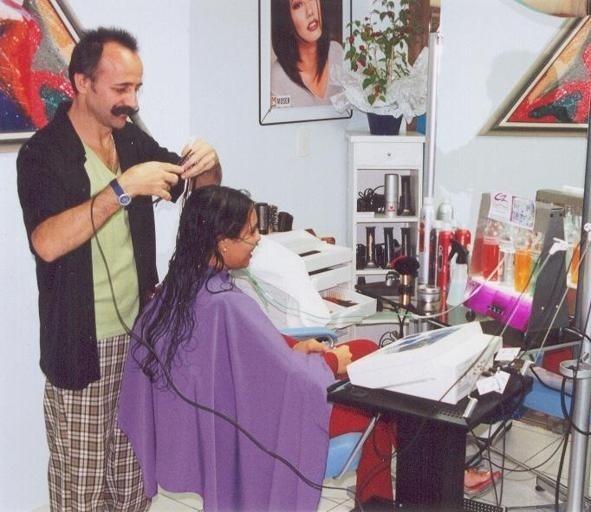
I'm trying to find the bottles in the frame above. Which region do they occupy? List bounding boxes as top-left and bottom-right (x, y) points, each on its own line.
(481, 220), (544, 293)
(563, 206), (581, 285)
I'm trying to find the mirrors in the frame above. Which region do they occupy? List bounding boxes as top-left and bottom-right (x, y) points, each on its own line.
(427, 1), (591, 328)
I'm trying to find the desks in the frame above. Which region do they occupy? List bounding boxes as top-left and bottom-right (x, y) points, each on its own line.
(327, 366), (534, 512)
(356, 277), (591, 512)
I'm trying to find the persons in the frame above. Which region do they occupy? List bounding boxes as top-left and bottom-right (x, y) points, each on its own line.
(116, 184), (500, 512)
(270, 0), (343, 109)
(16, 26), (222, 512)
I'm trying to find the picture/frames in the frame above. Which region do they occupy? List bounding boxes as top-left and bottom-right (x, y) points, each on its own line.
(257, 0), (353, 126)
(477, 15), (590, 137)
(0, 1), (84, 144)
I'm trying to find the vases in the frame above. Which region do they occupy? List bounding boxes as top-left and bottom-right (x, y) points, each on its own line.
(363, 106), (405, 135)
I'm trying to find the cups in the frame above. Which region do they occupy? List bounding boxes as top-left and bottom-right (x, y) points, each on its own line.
(255, 202), (268, 234)
(385, 173), (399, 216)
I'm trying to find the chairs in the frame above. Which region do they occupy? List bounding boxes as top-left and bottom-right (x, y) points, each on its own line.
(134, 315), (364, 512)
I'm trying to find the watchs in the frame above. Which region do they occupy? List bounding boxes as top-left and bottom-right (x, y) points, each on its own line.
(109, 179), (132, 207)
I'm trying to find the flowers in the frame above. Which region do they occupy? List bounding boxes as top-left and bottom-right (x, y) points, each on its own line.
(344, 1), (425, 105)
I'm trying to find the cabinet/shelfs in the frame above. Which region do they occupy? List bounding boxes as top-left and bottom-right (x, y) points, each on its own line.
(343, 133), (425, 324)
